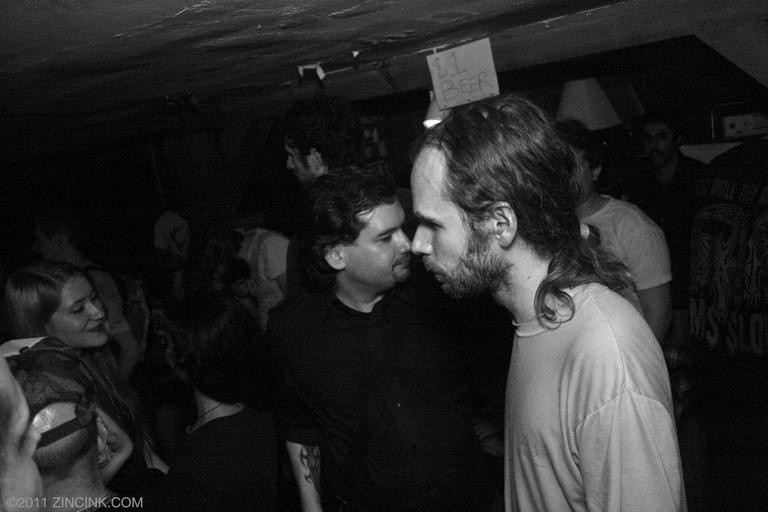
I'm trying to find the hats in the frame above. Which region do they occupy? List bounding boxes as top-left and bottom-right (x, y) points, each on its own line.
(0, 334), (100, 449)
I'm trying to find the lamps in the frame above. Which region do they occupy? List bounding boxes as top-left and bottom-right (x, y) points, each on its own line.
(555, 77), (621, 136)
